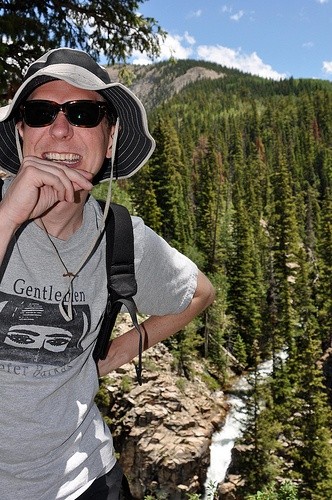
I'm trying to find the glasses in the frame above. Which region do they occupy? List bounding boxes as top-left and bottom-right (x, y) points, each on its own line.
(14, 99), (115, 127)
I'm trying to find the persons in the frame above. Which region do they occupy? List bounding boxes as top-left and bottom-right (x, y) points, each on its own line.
(0, 47), (215, 500)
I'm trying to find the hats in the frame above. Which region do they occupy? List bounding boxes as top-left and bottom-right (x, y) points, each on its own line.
(0, 47), (156, 184)
